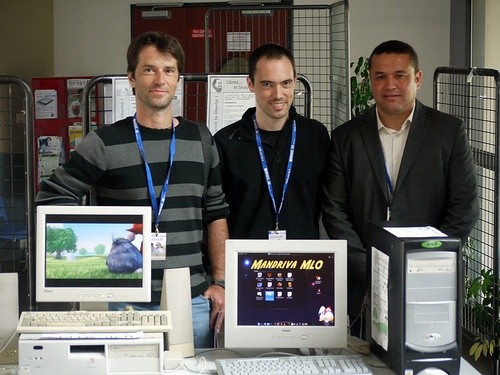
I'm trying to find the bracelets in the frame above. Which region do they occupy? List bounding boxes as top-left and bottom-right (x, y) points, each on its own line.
(212, 279), (224, 288)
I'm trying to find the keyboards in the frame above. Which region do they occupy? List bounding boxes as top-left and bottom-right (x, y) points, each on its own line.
(215, 354), (373, 375)
(17, 310), (172, 334)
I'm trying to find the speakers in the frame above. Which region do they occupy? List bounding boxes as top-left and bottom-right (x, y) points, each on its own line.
(0, 272), (19, 365)
(160, 266), (195, 358)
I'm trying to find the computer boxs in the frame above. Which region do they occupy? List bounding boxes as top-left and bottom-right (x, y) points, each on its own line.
(366, 220), (463, 375)
(18, 334), (164, 375)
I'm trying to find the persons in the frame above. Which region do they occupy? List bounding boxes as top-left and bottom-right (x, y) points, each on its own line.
(71, 89), (83, 117)
(155, 242), (165, 252)
(323, 40), (480, 341)
(213, 43), (331, 239)
(35, 30), (229, 349)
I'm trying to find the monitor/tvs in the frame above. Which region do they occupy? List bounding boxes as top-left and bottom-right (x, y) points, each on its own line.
(36, 205), (152, 311)
(224, 239), (347, 356)
